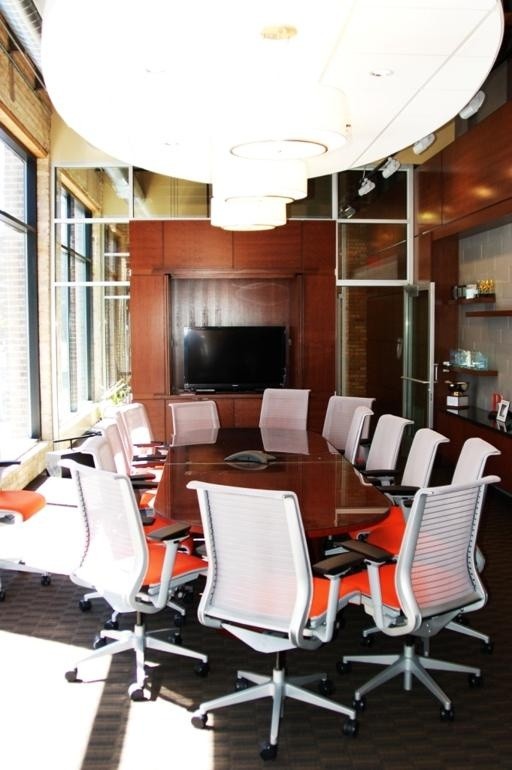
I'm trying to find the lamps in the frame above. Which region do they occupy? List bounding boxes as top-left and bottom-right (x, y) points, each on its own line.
(33, 1), (505, 232)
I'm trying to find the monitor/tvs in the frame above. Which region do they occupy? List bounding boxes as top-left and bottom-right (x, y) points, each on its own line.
(183, 325), (289, 389)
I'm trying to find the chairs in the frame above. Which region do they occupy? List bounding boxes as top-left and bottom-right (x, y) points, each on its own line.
(1, 388), (501, 656)
(186, 480), (358, 762)
(333, 475), (500, 737)
(66, 458), (209, 701)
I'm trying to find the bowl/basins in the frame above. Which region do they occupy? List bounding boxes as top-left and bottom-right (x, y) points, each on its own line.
(446, 382), (469, 393)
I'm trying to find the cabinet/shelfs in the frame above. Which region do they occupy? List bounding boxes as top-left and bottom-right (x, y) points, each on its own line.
(419, 198), (512, 499)
